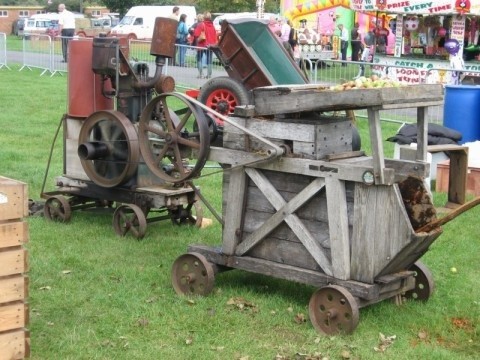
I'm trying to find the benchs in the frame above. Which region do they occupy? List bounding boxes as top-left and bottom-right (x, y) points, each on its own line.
(394, 143), (468, 204)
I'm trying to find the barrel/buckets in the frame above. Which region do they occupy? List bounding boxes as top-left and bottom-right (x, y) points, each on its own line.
(442, 85), (480, 145)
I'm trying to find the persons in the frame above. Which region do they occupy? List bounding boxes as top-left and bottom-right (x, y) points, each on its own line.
(271, 16), (480, 79)
(58, 3), (76, 63)
(168, 6), (180, 66)
(193, 12), (218, 79)
(176, 14), (190, 67)
(188, 14), (208, 68)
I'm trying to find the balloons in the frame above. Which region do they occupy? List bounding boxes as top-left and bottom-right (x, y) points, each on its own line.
(364, 16), (480, 56)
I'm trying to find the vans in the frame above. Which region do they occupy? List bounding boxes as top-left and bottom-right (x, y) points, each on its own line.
(16, 18), (60, 40)
(213, 12), (283, 46)
(90, 14), (118, 36)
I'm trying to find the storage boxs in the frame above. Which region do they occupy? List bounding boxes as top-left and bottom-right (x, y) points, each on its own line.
(436, 159), (480, 199)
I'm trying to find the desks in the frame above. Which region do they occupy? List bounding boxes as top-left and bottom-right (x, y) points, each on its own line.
(411, 45), (426, 59)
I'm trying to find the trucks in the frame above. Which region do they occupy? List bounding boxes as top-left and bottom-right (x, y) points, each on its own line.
(113, 6), (196, 44)
